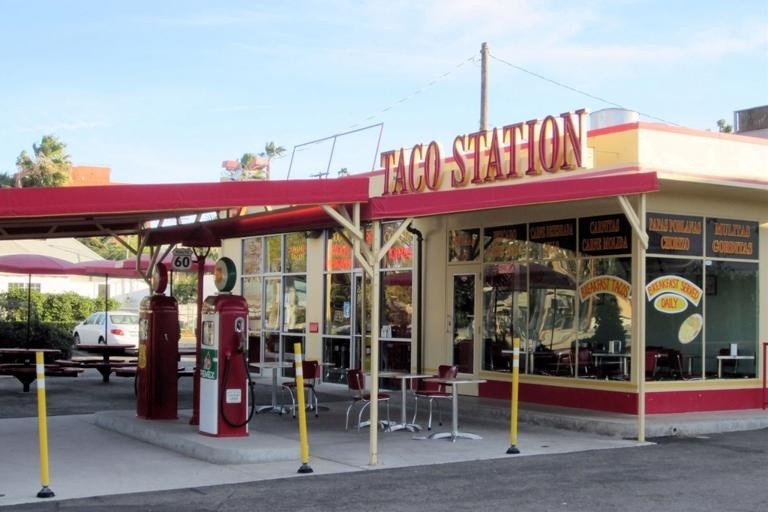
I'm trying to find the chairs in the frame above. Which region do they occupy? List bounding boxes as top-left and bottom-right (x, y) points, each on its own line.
(455, 338), (738, 382)
(280, 361), (319, 418)
(410, 365), (459, 431)
(344, 369), (392, 432)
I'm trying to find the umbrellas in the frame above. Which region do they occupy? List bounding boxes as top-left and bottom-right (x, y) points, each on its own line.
(0, 252), (87, 303)
(116, 245), (217, 288)
(72, 256), (150, 302)
(382, 257), (577, 311)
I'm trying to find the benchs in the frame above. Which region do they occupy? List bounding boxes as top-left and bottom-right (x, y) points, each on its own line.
(0, 356), (196, 392)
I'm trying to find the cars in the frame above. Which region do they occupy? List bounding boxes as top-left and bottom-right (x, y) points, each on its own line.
(72, 308), (144, 357)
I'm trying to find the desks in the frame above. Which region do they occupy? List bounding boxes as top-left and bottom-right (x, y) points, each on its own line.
(249, 361), (293, 415)
(76, 344), (136, 383)
(369, 372), (435, 433)
(0, 349), (62, 392)
(422, 378), (487, 443)
(717, 356), (755, 378)
